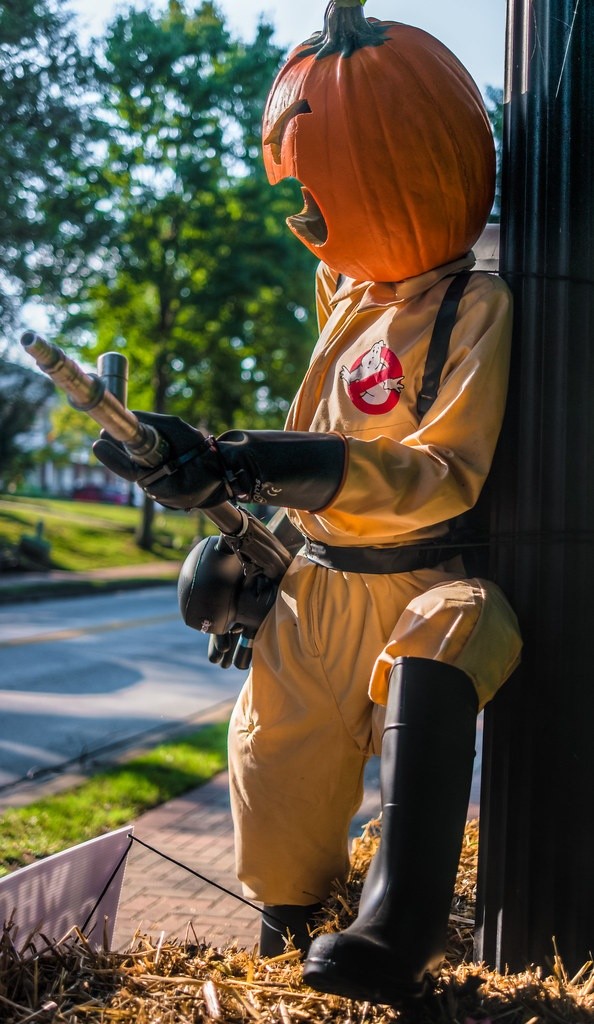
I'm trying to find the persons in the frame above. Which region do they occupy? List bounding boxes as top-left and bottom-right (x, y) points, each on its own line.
(88, 0), (522, 1002)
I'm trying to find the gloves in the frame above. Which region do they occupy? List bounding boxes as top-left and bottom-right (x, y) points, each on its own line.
(207, 509), (306, 671)
(93, 411), (349, 516)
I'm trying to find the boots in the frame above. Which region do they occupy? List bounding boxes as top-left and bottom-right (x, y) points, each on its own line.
(262, 903), (314, 958)
(303, 656), (479, 986)
